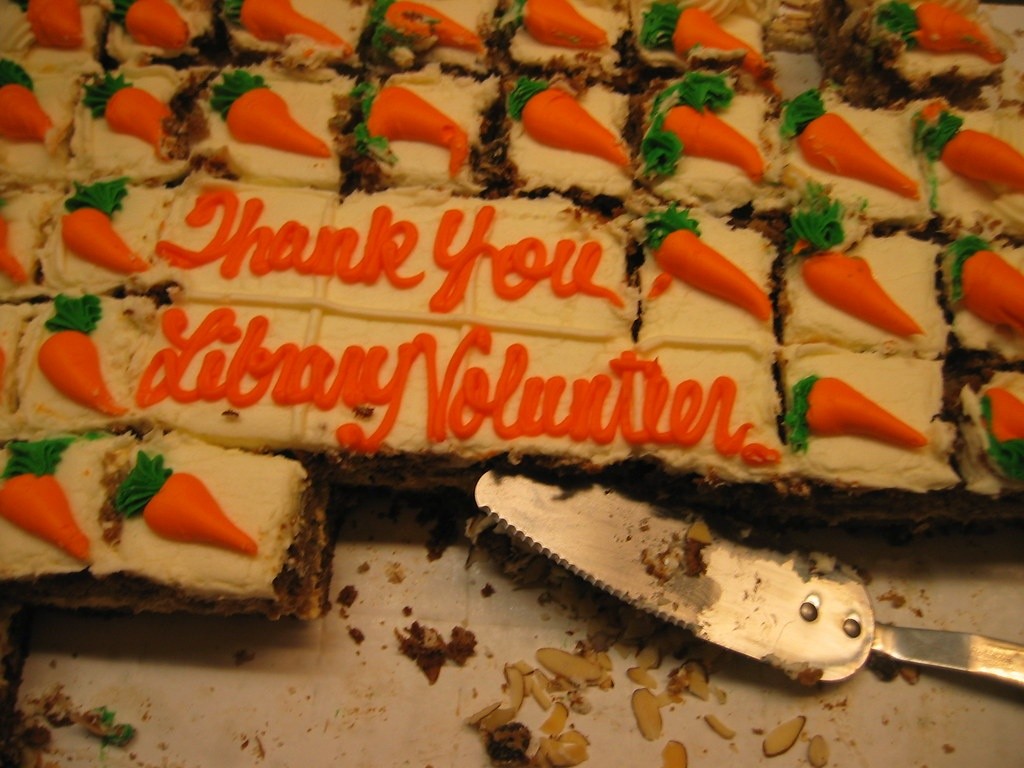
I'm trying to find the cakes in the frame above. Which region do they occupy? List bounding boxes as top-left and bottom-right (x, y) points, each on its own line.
(0, 0), (1023, 621)
